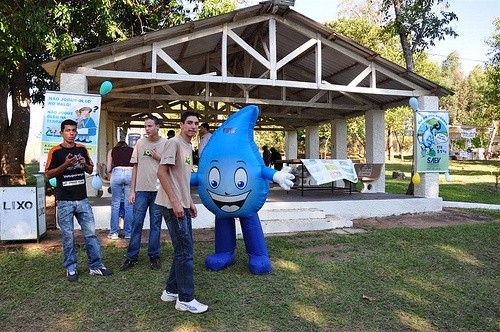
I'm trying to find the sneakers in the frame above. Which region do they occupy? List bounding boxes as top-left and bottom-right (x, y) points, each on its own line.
(160, 289), (177, 302)
(151, 257), (161, 269)
(174, 299), (208, 312)
(119, 257), (136, 270)
(89, 268), (112, 276)
(108, 232), (119, 241)
(66, 271), (79, 283)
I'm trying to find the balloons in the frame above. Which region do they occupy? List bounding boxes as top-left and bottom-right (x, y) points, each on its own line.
(408, 97), (418, 111)
(445, 173), (451, 182)
(49, 177), (57, 187)
(99, 80), (113, 96)
(92, 175), (102, 190)
(412, 173), (421, 185)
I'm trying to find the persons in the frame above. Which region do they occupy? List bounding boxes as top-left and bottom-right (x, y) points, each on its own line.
(167, 130), (175, 139)
(106, 141), (135, 240)
(45, 119), (113, 282)
(120, 116), (169, 273)
(198, 122), (213, 160)
(154, 112), (209, 315)
(270, 147), (283, 184)
(263, 146), (270, 167)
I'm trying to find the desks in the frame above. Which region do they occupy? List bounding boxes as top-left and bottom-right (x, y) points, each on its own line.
(275, 159), (360, 196)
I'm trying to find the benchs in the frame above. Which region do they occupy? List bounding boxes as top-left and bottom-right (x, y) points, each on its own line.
(352, 164), (383, 192)
